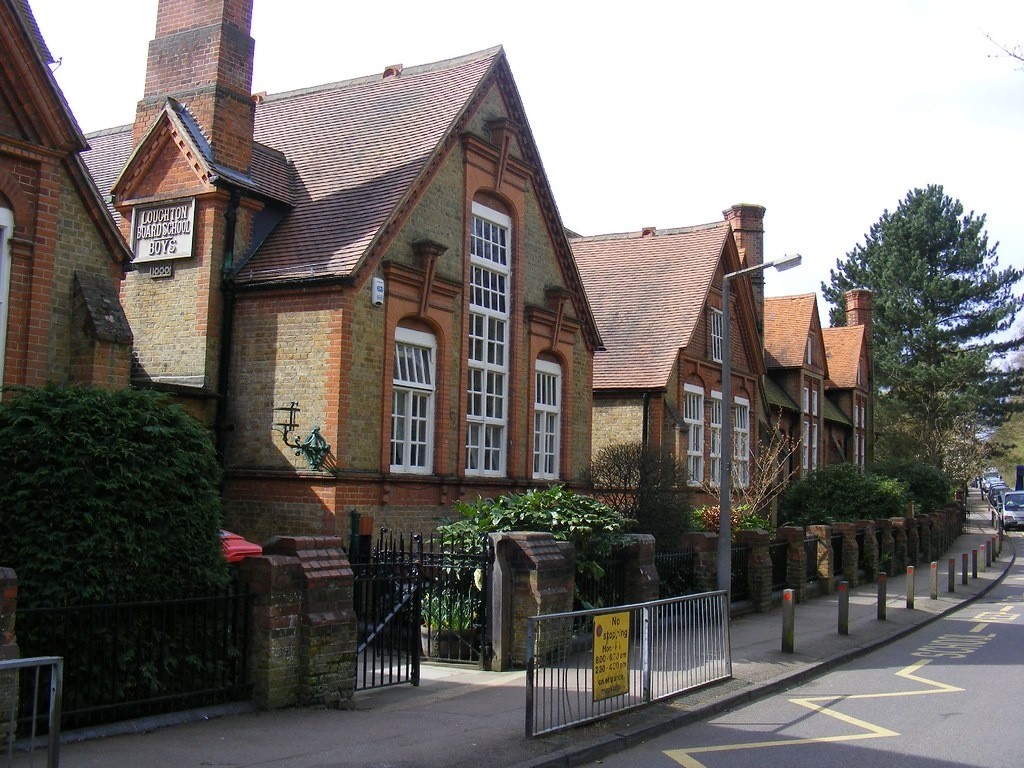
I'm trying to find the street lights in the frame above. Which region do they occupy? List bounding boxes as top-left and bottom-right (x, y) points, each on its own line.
(715, 250), (806, 625)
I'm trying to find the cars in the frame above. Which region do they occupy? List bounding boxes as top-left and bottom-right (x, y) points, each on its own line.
(978, 466), (1024, 531)
